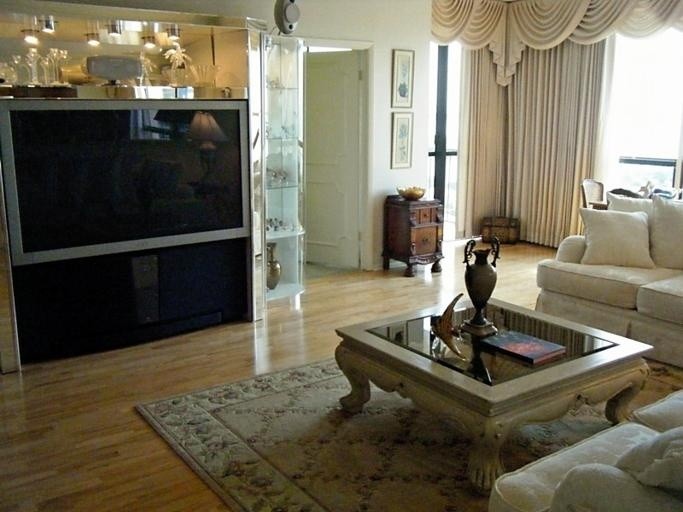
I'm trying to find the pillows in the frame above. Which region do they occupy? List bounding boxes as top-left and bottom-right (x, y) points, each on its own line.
(579, 191), (683, 269)
(613, 425), (683, 493)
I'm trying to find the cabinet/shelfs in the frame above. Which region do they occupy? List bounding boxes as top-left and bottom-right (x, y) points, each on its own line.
(381, 195), (443, 276)
(11, 236), (252, 365)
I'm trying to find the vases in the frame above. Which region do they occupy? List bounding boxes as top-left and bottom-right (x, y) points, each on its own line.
(267, 242), (281, 289)
(458, 235), (501, 337)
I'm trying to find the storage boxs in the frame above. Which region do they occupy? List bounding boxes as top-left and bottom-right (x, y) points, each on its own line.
(480, 216), (520, 244)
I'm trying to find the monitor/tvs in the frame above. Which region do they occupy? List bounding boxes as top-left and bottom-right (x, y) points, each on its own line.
(0, 96), (252, 267)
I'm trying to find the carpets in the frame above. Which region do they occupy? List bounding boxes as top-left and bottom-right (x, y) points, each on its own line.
(133, 356), (683, 509)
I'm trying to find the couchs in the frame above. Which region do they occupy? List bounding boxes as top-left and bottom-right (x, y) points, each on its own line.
(532, 194), (683, 372)
(488, 390), (683, 511)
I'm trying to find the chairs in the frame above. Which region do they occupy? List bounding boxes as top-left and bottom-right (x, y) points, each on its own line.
(581, 179), (604, 208)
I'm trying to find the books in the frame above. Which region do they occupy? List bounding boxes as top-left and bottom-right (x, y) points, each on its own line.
(481, 328), (566, 365)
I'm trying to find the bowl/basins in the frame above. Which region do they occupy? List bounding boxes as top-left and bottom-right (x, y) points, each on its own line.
(396, 186), (425, 201)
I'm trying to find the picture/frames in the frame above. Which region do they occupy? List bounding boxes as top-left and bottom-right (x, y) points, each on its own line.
(390, 48), (415, 170)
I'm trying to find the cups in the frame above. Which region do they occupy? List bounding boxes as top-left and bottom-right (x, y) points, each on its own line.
(0, 46), (71, 88)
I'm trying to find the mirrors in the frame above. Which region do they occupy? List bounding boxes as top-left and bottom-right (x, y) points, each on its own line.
(0, 35), (214, 89)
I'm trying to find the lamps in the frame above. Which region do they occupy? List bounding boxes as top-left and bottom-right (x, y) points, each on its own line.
(38, 16), (59, 32)
(87, 33), (100, 46)
(167, 29), (180, 40)
(108, 25), (122, 36)
(144, 38), (155, 48)
(20, 29), (40, 45)
(187, 111), (228, 153)
